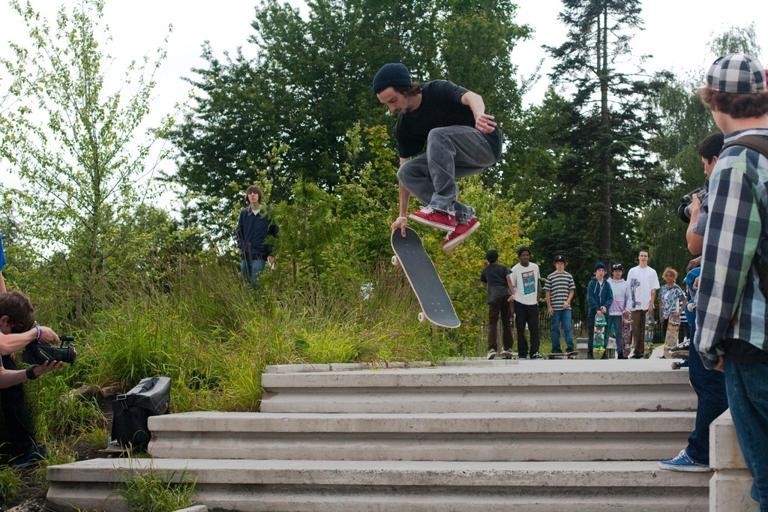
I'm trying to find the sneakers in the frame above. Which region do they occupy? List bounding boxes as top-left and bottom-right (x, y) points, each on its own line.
(409, 207), (481, 250)
(659, 449), (711, 472)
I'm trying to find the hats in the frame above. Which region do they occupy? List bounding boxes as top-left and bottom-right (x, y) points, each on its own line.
(554, 255), (565, 262)
(707, 55), (766, 94)
(595, 263), (624, 271)
(373, 63), (411, 89)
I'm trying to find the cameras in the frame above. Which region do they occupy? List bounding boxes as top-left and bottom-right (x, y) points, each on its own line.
(677, 188), (702, 224)
(21, 335), (77, 365)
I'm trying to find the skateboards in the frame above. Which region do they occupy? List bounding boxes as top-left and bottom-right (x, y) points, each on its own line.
(546, 350), (579, 359)
(672, 350), (689, 370)
(593, 312), (608, 358)
(499, 352), (518, 360)
(391, 227), (461, 327)
(621, 310), (634, 357)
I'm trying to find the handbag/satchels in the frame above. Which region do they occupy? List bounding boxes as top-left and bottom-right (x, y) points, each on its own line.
(111, 376), (170, 452)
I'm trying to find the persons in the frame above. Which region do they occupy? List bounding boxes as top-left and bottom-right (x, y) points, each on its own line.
(1, 291), (63, 390)
(237, 185), (279, 290)
(691, 52), (768, 512)
(659, 131), (728, 473)
(372, 63), (501, 252)
(0, 230), (44, 470)
(481, 248), (701, 367)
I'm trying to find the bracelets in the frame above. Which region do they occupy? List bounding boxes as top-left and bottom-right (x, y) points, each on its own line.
(26, 365), (38, 380)
(35, 325), (41, 341)
(396, 217), (408, 221)
